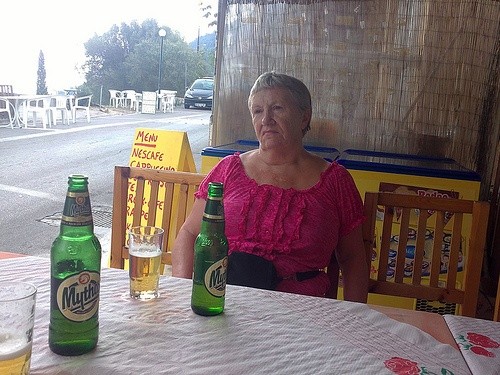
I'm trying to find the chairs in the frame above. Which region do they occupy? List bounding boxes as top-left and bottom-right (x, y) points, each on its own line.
(363, 191), (491, 319)
(0, 86), (177, 130)
(110, 166), (208, 275)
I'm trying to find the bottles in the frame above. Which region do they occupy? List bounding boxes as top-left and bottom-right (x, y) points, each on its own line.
(190, 181), (229, 317)
(48, 174), (101, 356)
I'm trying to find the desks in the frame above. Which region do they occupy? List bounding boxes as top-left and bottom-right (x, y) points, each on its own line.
(0, 95), (49, 130)
(50, 94), (74, 119)
(0, 252), (500, 375)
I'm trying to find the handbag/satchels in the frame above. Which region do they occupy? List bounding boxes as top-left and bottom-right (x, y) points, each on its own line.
(226, 252), (277, 292)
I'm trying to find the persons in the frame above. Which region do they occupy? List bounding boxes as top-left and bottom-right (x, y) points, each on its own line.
(170, 72), (368, 303)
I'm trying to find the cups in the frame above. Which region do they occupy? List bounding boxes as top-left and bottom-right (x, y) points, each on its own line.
(0, 281), (37, 375)
(127, 225), (164, 301)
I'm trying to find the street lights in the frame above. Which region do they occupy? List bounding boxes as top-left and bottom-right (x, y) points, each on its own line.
(157, 29), (166, 110)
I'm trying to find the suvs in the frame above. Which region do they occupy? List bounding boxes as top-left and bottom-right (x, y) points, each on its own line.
(184, 76), (214, 110)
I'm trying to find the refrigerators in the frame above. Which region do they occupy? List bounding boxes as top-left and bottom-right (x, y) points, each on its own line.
(200, 140), (481, 318)
